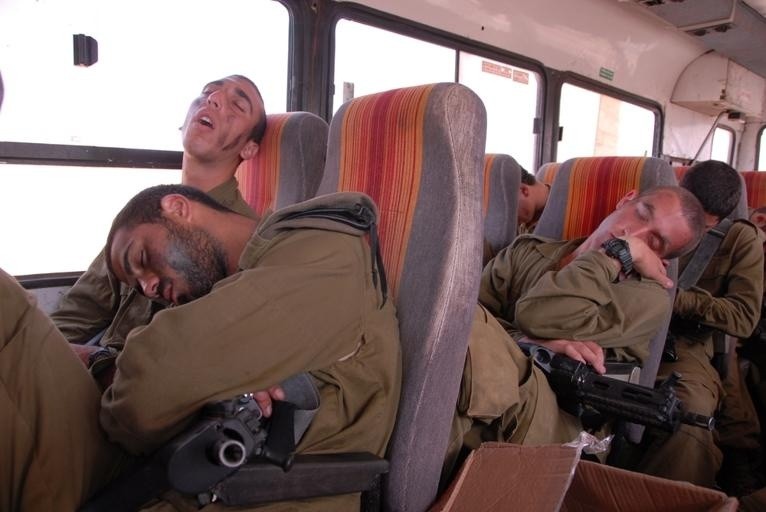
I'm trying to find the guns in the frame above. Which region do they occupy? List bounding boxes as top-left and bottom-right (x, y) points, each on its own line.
(517, 341), (716, 432)
(154, 391), (298, 493)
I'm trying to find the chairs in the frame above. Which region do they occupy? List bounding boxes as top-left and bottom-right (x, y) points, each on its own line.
(231, 109), (332, 212)
(480, 152), (522, 265)
(530, 157), (681, 388)
(740, 170), (765, 216)
(670, 167), (749, 229)
(534, 162), (562, 186)
(201, 83), (488, 509)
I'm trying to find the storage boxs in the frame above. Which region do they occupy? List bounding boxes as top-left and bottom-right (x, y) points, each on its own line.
(430, 440), (740, 511)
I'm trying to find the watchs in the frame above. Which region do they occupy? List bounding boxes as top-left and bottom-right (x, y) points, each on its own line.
(88, 346), (109, 370)
(602, 238), (634, 277)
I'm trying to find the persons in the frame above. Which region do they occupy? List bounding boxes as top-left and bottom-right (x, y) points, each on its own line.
(48, 73), (268, 386)
(641, 161), (765, 488)
(445, 186), (706, 480)
(518, 166), (552, 236)
(0, 65), (103, 510)
(97, 181), (404, 512)
